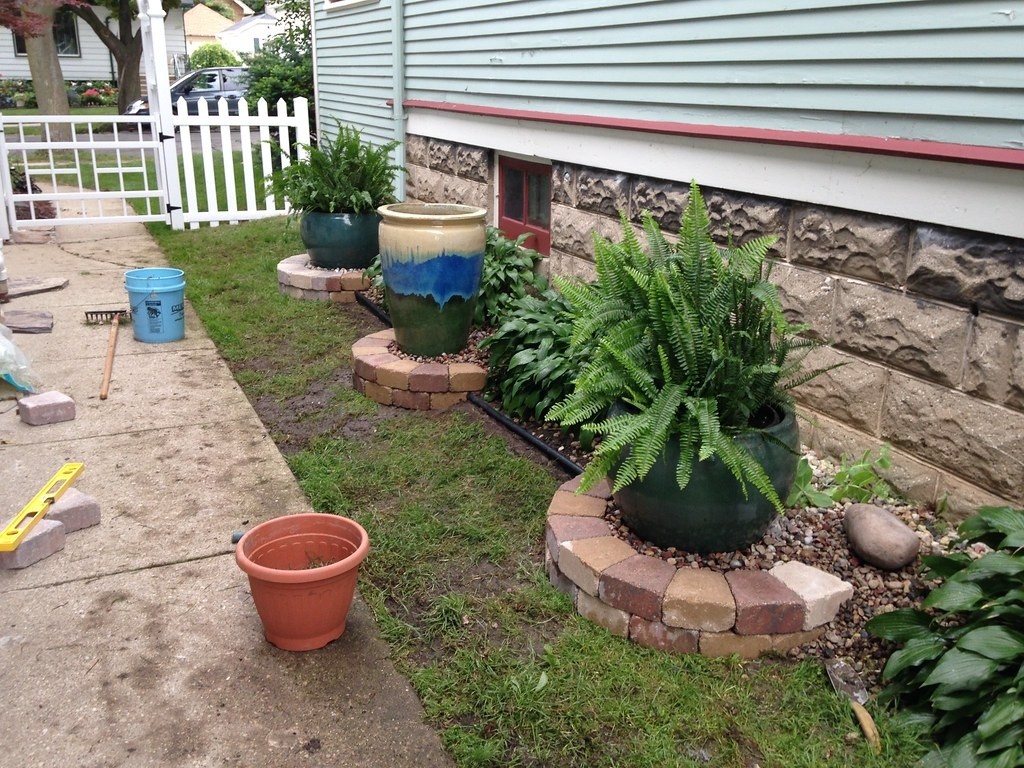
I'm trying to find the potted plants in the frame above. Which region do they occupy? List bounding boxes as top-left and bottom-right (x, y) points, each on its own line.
(12, 91), (30, 108)
(258, 113), (411, 268)
(554, 178), (852, 555)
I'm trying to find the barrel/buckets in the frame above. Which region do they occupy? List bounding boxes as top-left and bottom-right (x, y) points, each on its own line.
(123, 267), (186, 343)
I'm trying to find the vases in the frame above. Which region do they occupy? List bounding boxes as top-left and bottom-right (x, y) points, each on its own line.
(376, 202), (487, 357)
(235, 512), (370, 651)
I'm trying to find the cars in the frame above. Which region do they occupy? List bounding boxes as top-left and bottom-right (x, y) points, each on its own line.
(121, 65), (256, 135)
